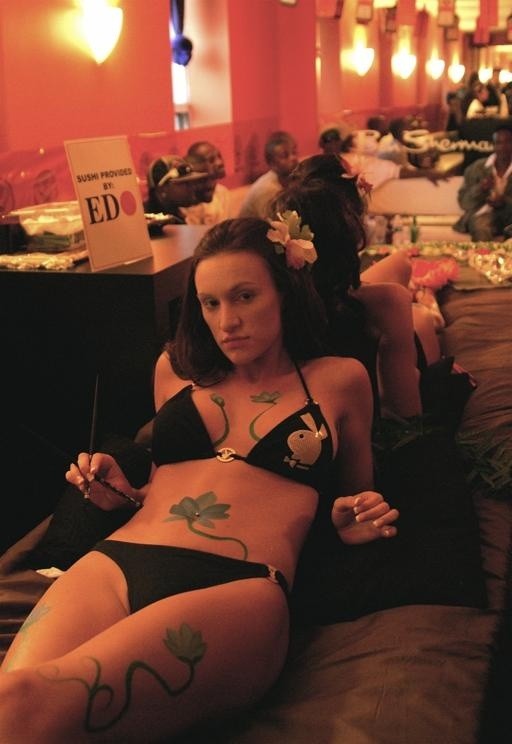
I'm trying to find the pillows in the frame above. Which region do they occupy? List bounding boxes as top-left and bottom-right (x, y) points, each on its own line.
(29, 433), (485, 616)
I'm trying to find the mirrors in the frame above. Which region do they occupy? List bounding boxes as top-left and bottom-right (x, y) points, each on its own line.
(0, 2), (511, 496)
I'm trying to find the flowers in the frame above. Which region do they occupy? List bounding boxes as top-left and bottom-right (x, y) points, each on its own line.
(334, 150), (374, 200)
(263, 209), (319, 272)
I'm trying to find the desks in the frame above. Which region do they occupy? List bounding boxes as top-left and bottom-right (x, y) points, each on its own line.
(0, 223), (218, 357)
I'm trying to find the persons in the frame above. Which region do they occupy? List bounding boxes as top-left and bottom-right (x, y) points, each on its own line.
(4, 216), (401, 742)
(176, 139), (235, 225)
(235, 127), (301, 223)
(320, 71), (511, 180)
(456, 125), (511, 243)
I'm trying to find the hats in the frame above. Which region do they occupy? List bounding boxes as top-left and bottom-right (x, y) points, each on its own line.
(147, 153), (209, 189)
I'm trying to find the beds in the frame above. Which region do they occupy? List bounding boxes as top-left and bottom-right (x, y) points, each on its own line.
(1, 568), (497, 744)
(12, 242), (511, 612)
